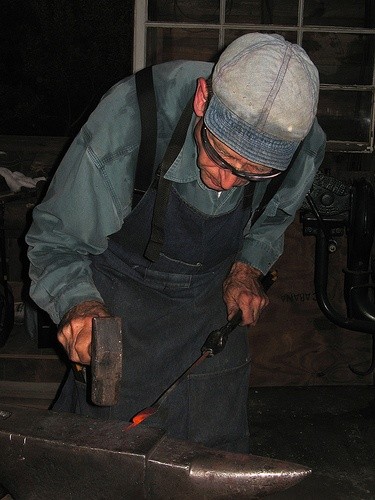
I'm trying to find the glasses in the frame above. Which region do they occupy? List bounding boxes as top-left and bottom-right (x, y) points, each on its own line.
(201, 123), (283, 182)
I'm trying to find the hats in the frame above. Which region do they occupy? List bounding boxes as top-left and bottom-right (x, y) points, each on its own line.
(205, 33), (319, 171)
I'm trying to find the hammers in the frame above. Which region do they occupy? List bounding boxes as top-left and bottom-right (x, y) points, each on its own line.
(74, 316), (122, 407)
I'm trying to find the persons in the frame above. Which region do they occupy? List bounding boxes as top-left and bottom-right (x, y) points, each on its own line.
(26, 31), (326, 455)
